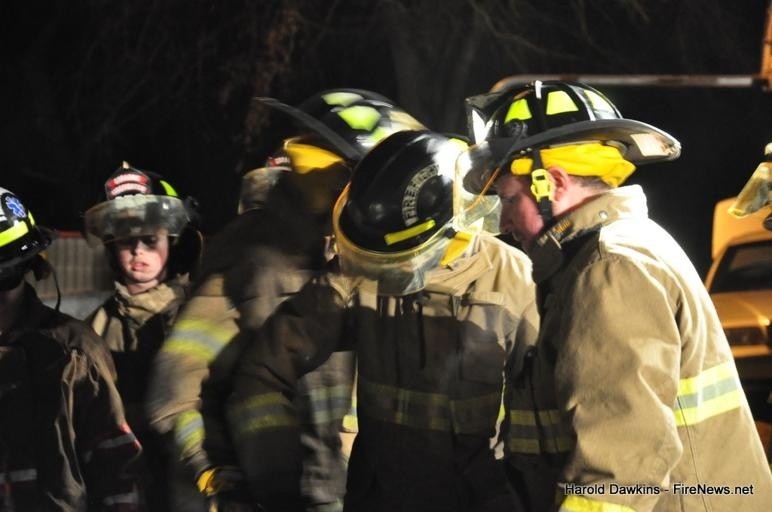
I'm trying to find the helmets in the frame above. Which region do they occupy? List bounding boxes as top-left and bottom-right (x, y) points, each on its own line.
(0, 187), (56, 282)
(460, 79), (680, 197)
(330, 125), (503, 270)
(262, 87), (434, 178)
(80, 164), (192, 240)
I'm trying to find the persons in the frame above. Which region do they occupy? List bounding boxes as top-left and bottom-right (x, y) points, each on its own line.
(0, 73), (770, 512)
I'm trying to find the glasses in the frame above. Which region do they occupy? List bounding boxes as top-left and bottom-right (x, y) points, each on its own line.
(0, 271), (26, 291)
